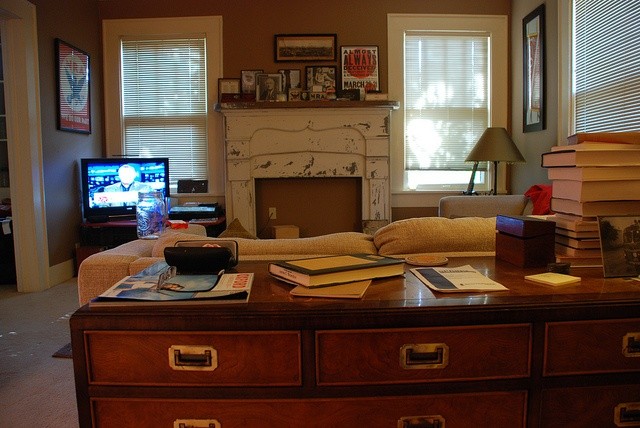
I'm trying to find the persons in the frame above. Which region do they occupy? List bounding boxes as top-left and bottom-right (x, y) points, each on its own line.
(104, 165), (152, 191)
(260, 78), (280, 100)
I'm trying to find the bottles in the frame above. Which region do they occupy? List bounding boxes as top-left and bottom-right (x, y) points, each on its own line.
(136, 191), (165, 239)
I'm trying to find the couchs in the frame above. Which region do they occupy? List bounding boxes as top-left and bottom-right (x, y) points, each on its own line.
(78, 192), (555, 307)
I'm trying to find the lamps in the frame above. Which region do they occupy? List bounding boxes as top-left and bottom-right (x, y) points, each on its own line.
(465, 127), (525, 196)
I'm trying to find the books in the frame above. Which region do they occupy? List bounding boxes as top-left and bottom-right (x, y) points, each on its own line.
(267, 253), (408, 289)
(96, 272), (255, 303)
(540, 129), (640, 278)
(365, 92), (388, 102)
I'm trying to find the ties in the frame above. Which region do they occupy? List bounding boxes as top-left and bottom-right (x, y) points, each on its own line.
(122, 185), (130, 207)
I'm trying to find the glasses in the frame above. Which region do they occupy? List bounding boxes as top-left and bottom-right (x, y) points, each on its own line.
(156, 266), (227, 293)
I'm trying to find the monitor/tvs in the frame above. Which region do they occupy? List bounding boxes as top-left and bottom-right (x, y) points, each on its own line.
(79, 156), (170, 223)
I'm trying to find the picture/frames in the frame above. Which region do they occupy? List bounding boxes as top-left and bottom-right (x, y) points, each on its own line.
(321, 92), (326, 100)
(272, 34), (338, 62)
(53, 36), (92, 135)
(597, 213), (639, 277)
(254, 72), (282, 102)
(239, 69), (264, 102)
(278, 69), (301, 101)
(522, 2), (547, 134)
(340, 44), (379, 98)
(305, 66), (337, 99)
(217, 77), (241, 102)
(316, 92), (321, 100)
(288, 87), (301, 101)
(311, 92), (316, 100)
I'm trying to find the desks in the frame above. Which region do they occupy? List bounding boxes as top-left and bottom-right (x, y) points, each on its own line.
(72, 211), (226, 275)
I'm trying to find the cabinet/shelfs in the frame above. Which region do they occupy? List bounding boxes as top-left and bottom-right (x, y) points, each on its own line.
(72, 249), (538, 427)
(535, 269), (639, 427)
